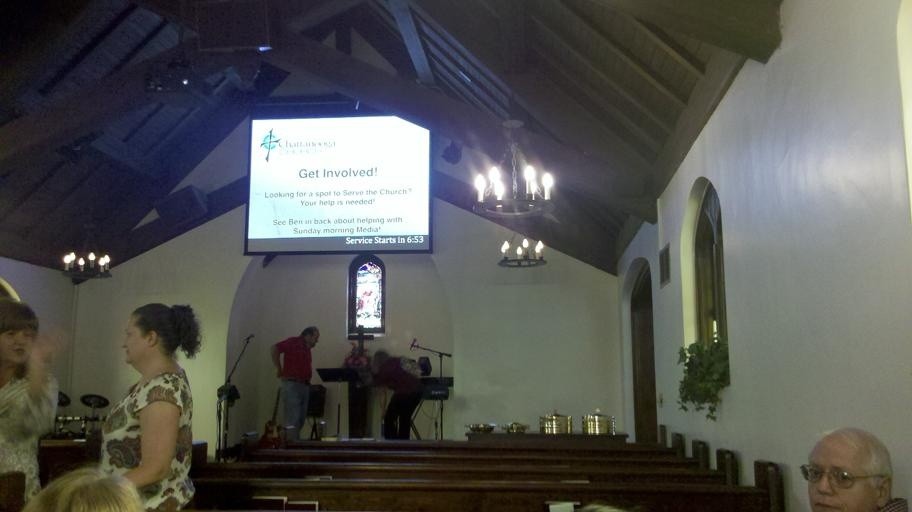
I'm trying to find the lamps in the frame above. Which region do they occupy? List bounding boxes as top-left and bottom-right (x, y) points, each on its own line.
(57, 204), (112, 279)
(470, 97), (559, 269)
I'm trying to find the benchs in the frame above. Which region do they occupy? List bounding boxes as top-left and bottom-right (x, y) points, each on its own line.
(190, 438), (771, 512)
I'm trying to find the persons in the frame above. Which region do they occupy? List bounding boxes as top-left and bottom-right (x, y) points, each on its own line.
(20, 463), (148, 512)
(371, 355), (428, 436)
(100, 302), (203, 512)
(0, 292), (60, 512)
(799, 427), (911, 512)
(269, 325), (319, 442)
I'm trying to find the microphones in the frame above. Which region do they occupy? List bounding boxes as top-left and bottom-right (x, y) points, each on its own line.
(243, 334), (255, 340)
(410, 338), (417, 351)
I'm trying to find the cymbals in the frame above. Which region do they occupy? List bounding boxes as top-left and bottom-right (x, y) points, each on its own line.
(58, 391), (71, 407)
(80, 394), (110, 409)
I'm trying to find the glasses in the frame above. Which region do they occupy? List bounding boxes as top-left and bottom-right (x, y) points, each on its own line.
(799, 464), (888, 489)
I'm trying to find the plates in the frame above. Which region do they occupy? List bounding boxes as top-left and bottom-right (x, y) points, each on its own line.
(465, 422), (496, 432)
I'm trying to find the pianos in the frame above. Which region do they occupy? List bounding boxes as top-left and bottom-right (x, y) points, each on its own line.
(418, 375), (453, 399)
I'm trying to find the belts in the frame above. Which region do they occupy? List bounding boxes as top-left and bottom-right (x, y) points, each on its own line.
(282, 379), (310, 385)
